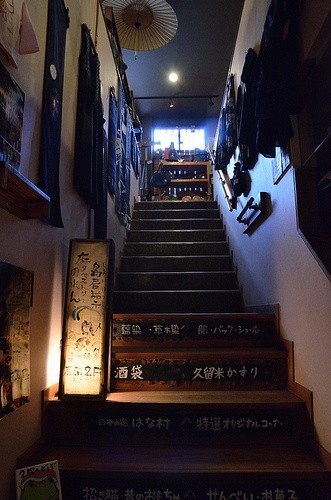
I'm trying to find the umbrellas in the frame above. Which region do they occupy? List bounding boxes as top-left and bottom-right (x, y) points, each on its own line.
(102, 0), (179, 60)
